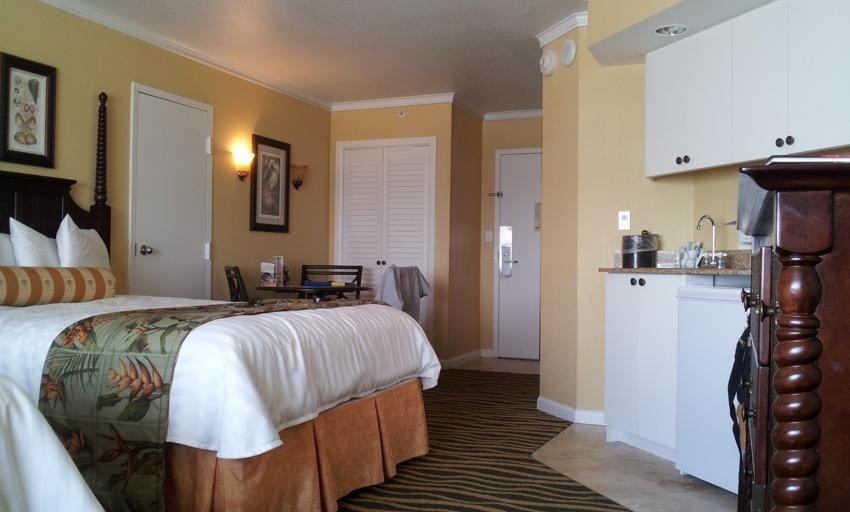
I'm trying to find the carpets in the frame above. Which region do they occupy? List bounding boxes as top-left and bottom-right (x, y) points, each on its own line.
(333, 366), (634, 512)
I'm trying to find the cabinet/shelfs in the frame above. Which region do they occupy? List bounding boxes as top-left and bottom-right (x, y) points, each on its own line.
(735, 157), (850, 510)
(642, 1), (850, 180)
(606, 274), (684, 444)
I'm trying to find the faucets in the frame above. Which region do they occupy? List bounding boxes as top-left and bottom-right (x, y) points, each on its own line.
(697, 213), (717, 268)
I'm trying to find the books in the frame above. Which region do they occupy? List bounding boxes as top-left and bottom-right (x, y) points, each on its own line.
(330, 281), (350, 287)
(304, 281), (336, 286)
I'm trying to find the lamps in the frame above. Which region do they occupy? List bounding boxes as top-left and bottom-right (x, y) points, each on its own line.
(290, 163), (308, 191)
(230, 141), (256, 182)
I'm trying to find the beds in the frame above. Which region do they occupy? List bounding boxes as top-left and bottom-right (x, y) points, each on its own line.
(1, 170), (433, 510)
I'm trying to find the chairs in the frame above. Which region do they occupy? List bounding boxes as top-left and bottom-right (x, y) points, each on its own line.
(224, 264), (424, 323)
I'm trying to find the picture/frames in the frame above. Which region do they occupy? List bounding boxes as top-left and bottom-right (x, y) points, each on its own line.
(0, 50), (58, 168)
(249, 132), (291, 234)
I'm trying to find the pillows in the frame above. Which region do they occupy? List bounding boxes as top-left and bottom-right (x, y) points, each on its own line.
(1, 212), (120, 309)
